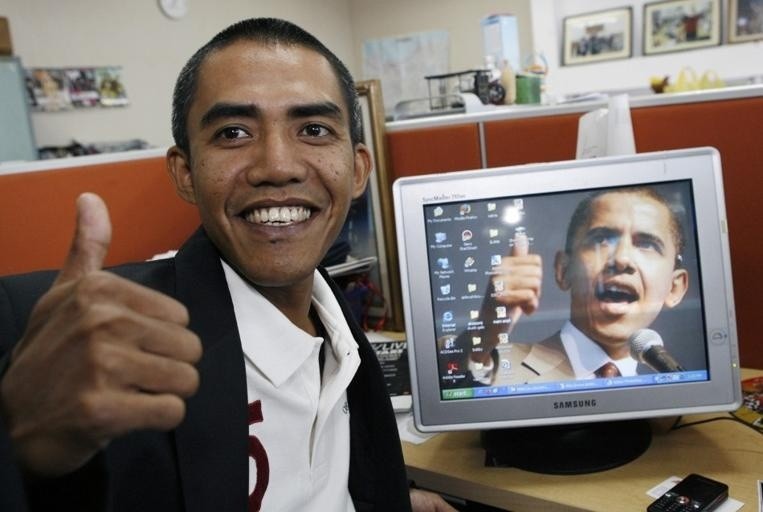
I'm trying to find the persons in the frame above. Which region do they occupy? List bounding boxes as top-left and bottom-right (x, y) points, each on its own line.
(436, 189), (688, 388)
(1, 20), (456, 512)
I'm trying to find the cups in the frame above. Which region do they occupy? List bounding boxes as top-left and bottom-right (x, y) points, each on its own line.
(515, 78), (540, 105)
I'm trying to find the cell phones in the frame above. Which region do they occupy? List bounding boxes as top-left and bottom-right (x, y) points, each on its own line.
(644, 473), (727, 512)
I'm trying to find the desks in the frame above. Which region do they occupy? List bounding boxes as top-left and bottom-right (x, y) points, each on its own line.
(368, 333), (762, 512)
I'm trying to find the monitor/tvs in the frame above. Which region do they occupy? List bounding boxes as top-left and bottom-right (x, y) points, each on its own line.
(391, 145), (743, 474)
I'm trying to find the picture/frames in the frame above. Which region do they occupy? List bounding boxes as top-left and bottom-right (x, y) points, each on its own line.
(322, 78), (401, 332)
(563, 0), (762, 66)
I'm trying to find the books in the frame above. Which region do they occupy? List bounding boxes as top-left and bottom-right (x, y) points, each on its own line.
(26, 68), (126, 110)
